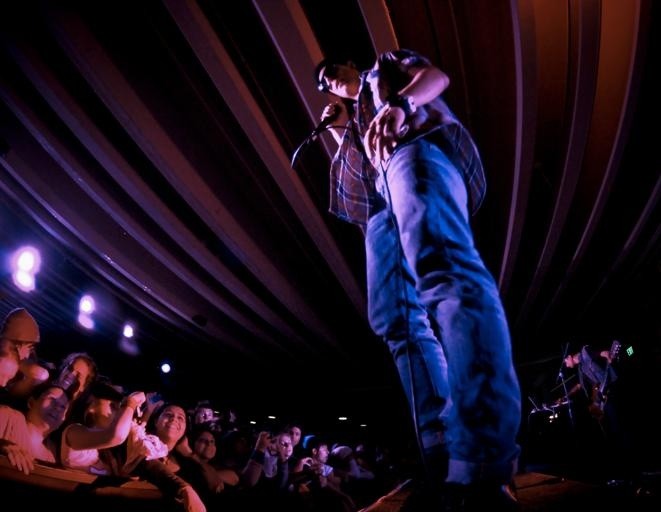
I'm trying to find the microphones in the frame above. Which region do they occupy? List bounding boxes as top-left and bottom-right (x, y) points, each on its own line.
(312, 103), (341, 139)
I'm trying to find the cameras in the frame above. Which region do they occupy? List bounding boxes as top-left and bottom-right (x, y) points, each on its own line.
(136, 403), (148, 417)
(320, 466), (333, 477)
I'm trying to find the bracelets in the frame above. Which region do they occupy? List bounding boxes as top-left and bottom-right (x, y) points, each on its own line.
(381, 90), (418, 128)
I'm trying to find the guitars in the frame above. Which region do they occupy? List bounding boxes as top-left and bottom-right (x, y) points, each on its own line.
(588, 340), (621, 419)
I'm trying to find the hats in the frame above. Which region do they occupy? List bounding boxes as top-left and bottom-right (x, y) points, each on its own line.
(3, 307), (40, 343)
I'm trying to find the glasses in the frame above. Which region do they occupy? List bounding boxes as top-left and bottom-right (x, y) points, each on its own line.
(316, 62), (337, 94)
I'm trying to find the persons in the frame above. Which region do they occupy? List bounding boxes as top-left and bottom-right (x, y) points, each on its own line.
(2, 305), (391, 511)
(307, 40), (529, 511)
(553, 339), (628, 487)
